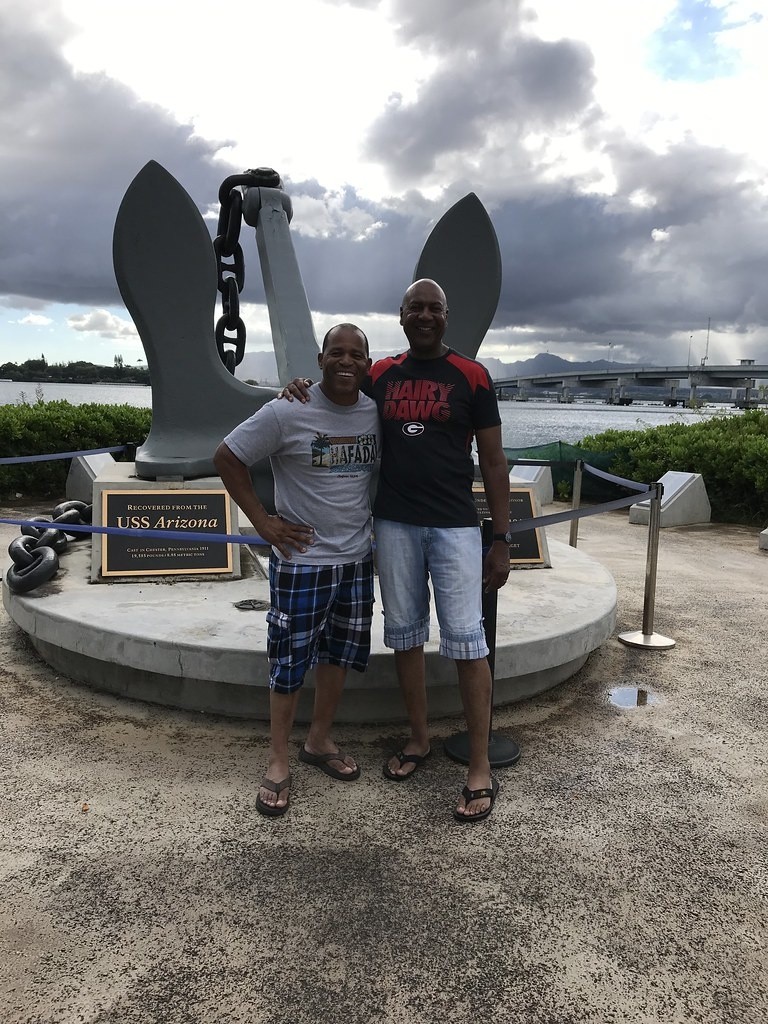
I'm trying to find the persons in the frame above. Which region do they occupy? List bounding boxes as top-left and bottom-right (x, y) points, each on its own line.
(213, 324), (382, 817)
(278, 278), (511, 821)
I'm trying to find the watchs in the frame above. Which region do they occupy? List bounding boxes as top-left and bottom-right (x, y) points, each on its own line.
(493, 532), (511, 543)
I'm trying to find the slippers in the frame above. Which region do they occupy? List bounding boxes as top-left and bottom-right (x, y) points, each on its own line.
(297, 739), (361, 780)
(382, 742), (429, 781)
(256, 771), (292, 815)
(453, 775), (498, 822)
